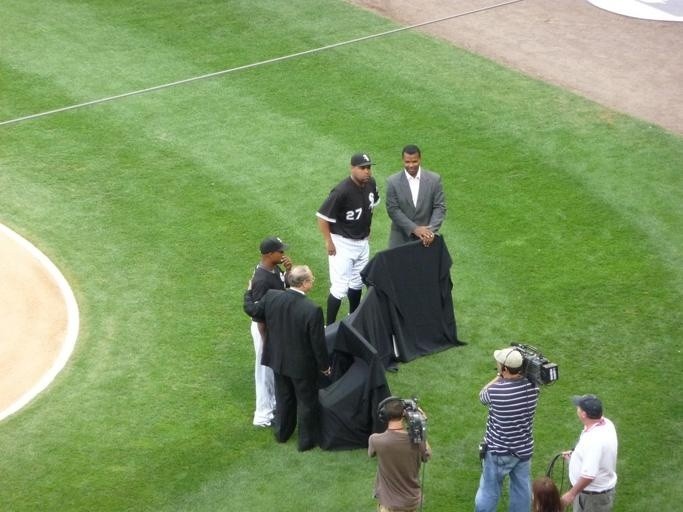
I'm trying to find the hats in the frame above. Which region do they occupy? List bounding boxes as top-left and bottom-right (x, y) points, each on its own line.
(351, 153), (376, 167)
(574, 394), (602, 419)
(494, 348), (523, 368)
(260, 235), (290, 253)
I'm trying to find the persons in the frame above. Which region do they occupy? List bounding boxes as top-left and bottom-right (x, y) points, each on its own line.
(559, 393), (618, 512)
(527, 477), (564, 512)
(384, 146), (448, 248)
(472, 347), (538, 512)
(242, 267), (331, 451)
(248, 237), (292, 427)
(366, 398), (431, 512)
(313, 152), (381, 326)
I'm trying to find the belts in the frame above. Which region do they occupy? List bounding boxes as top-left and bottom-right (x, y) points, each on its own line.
(581, 489), (613, 495)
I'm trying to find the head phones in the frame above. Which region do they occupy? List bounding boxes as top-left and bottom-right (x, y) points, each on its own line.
(376, 396), (407, 425)
(502, 348), (522, 372)
